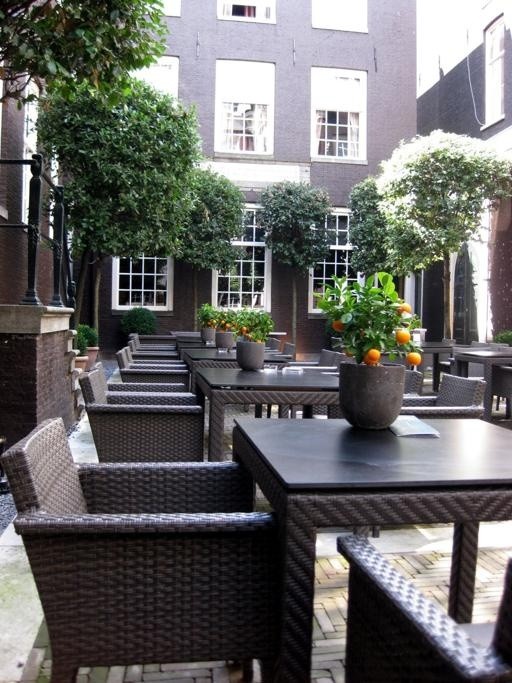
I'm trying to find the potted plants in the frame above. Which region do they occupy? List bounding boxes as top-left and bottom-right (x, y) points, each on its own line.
(77, 323), (100, 372)
(235, 305), (275, 370)
(214, 309), (238, 348)
(197, 303), (220, 343)
(312, 271), (422, 429)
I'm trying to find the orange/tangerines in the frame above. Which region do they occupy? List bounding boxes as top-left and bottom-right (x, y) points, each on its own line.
(363, 354), (378, 365)
(201, 314), (261, 343)
(332, 321), (344, 332)
(394, 329), (411, 343)
(346, 350), (355, 357)
(367, 348), (381, 361)
(406, 352), (421, 367)
(396, 303), (411, 315)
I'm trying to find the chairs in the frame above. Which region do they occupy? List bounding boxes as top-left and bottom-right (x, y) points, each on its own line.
(334, 526), (512, 681)
(263, 333), (346, 366)
(116, 332), (190, 384)
(79, 373), (206, 461)
(312, 332), (512, 437)
(1, 418), (275, 682)
(93, 364), (192, 396)
